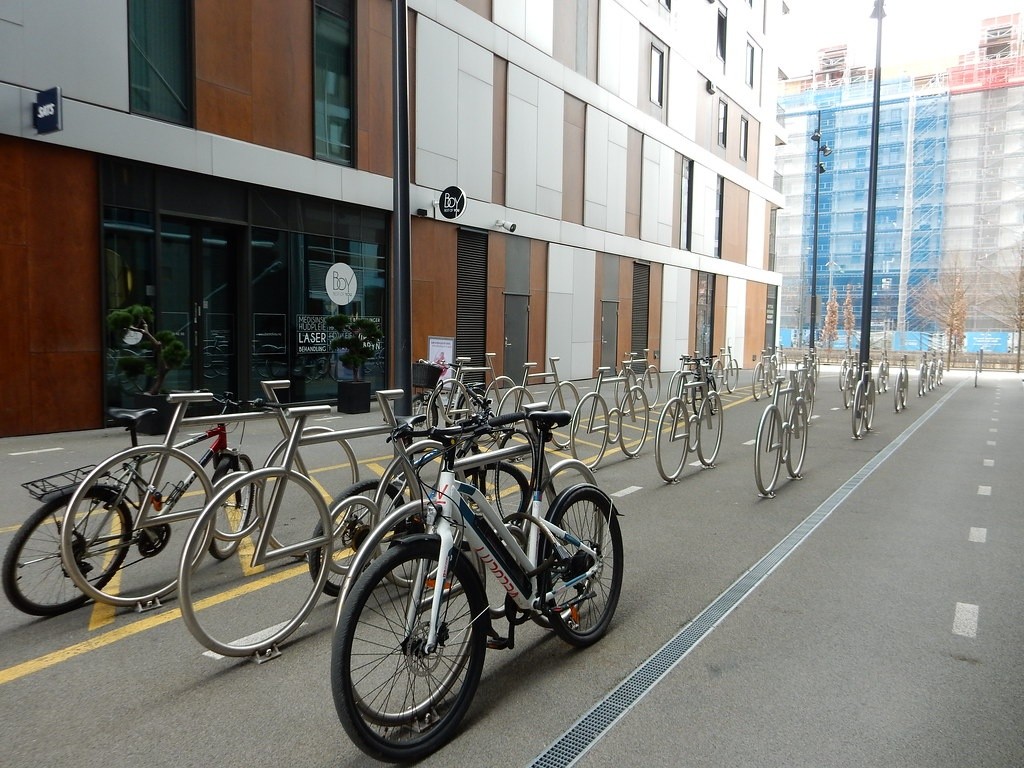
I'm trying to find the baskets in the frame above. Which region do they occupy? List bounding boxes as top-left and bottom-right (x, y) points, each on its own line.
(411, 362), (443, 389)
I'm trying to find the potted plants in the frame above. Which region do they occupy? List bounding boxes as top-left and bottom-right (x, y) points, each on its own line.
(325, 314), (383, 415)
(105, 305), (193, 435)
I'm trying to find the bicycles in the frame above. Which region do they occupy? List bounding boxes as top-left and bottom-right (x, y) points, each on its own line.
(54, 344), (982, 729)
(3, 390), (282, 614)
(332, 411), (623, 767)
